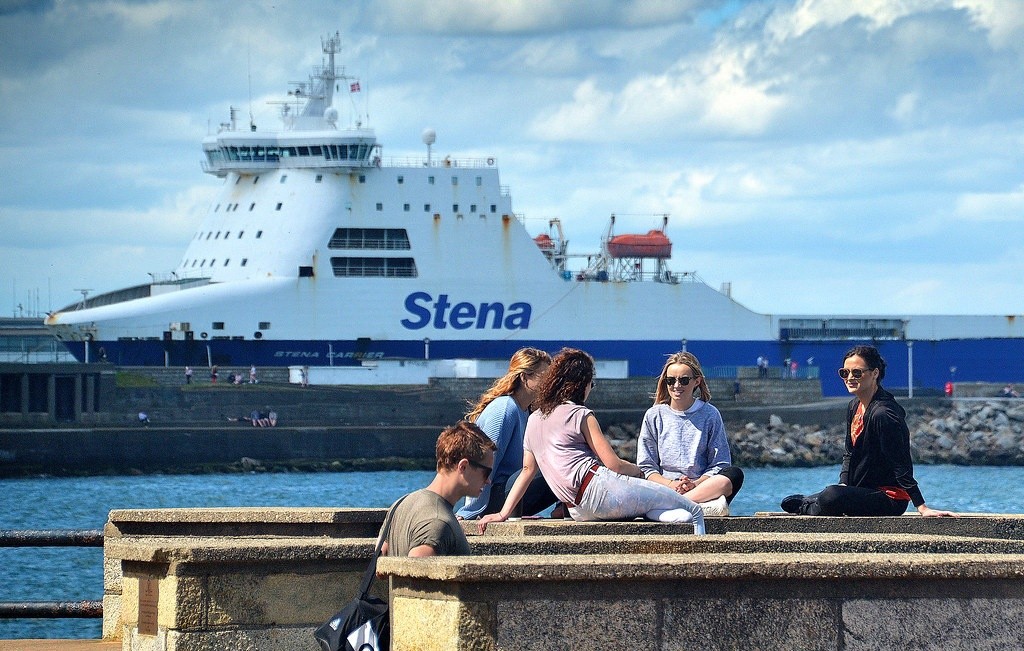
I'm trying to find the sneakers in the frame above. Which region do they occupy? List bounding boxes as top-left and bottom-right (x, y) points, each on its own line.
(699, 495), (729, 517)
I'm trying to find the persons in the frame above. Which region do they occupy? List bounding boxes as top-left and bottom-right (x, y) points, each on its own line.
(227, 372), (242, 385)
(185, 366), (192, 384)
(1004, 384), (1019, 397)
(453, 345), (570, 520)
(251, 408), (277, 427)
(139, 409), (149, 428)
(248, 364), (258, 384)
(783, 355), (796, 377)
(733, 379), (741, 401)
(99, 345), (106, 363)
(757, 356), (768, 378)
(375, 422), (498, 581)
(636, 351), (745, 517)
(477, 347), (705, 536)
(298, 368), (305, 387)
(781, 346), (962, 519)
(211, 365), (217, 382)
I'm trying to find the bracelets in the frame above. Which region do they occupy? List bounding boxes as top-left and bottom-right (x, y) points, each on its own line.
(668, 480), (673, 486)
(499, 512), (509, 521)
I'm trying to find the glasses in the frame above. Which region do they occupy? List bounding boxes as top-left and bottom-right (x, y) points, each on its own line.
(591, 380), (596, 390)
(665, 377), (693, 386)
(839, 368), (869, 380)
(469, 460), (492, 481)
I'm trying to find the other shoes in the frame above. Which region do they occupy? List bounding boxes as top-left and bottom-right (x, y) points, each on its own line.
(781, 495), (804, 514)
(551, 503), (564, 519)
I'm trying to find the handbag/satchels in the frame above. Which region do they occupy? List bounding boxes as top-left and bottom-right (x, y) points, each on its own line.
(314, 494), (406, 651)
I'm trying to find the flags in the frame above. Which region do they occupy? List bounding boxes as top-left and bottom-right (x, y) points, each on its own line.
(350, 82), (360, 93)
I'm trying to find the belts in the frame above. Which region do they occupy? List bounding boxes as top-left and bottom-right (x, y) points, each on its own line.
(565, 464), (599, 508)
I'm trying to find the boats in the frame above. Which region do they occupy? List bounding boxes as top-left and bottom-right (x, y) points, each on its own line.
(41, 25), (800, 389)
(606, 229), (673, 259)
